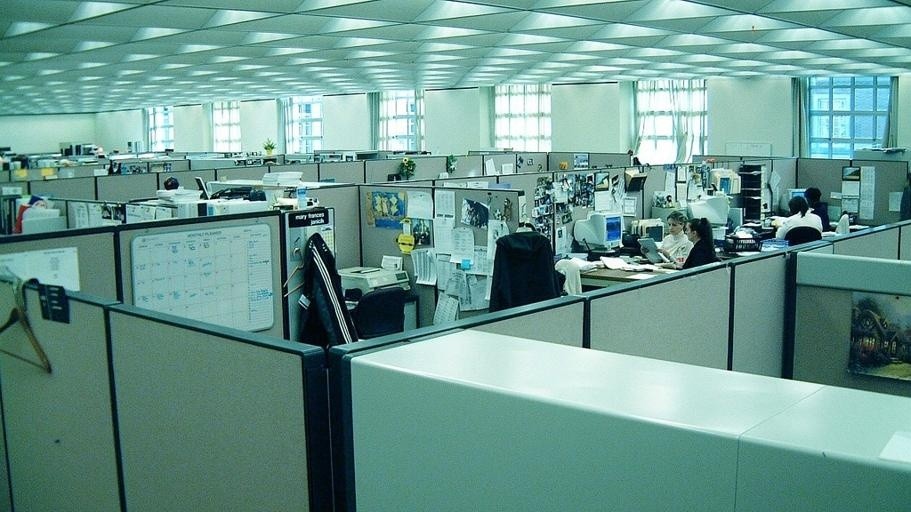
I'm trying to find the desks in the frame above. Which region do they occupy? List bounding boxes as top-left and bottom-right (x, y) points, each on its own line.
(577, 254), (680, 288)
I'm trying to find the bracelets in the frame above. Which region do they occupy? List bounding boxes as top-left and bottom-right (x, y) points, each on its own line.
(668, 253), (672, 260)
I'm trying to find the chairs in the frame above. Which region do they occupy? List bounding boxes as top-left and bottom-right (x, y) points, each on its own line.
(349, 286), (407, 339)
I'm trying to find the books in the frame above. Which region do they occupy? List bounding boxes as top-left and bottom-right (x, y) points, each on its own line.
(637, 237), (663, 263)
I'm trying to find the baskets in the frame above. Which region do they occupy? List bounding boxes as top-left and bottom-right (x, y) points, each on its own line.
(760, 239), (788, 249)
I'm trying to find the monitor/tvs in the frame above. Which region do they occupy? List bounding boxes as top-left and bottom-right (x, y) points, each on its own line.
(572, 213), (622, 248)
(781, 187), (806, 212)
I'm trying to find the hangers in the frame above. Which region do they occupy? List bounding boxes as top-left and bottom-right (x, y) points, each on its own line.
(0, 277), (53, 373)
(283, 247), (304, 296)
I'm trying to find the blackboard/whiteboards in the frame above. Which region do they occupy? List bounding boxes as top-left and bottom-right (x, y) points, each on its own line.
(724, 142), (772, 157)
(130, 223), (275, 333)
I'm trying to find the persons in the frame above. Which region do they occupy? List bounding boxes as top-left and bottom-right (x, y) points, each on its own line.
(662, 217), (717, 270)
(641, 210), (695, 270)
(416, 219), (430, 245)
(803, 187), (830, 231)
(101, 206), (111, 220)
(113, 206), (125, 223)
(901, 172), (911, 223)
(775, 195), (824, 240)
(412, 219), (422, 244)
